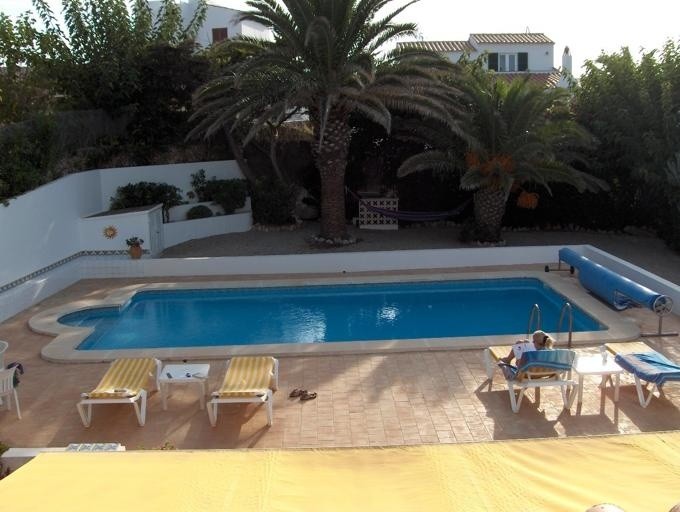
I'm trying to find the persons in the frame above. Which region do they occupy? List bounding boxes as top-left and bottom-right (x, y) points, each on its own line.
(499, 330), (553, 379)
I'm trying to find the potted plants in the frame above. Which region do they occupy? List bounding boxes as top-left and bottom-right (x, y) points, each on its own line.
(125, 236), (142, 259)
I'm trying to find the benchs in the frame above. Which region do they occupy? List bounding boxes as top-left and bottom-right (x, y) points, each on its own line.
(604, 341), (679, 409)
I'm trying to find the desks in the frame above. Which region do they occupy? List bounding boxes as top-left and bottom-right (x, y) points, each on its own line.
(156, 363), (212, 412)
(570, 354), (624, 406)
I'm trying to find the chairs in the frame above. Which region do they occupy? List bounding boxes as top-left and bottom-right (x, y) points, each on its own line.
(485, 340), (578, 410)
(0, 364), (24, 420)
(205, 356), (280, 427)
(76, 354), (160, 429)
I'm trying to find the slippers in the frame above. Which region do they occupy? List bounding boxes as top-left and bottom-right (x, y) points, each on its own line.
(290, 389), (317, 400)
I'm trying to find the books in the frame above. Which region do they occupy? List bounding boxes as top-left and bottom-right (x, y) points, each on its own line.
(512, 343), (535, 360)
